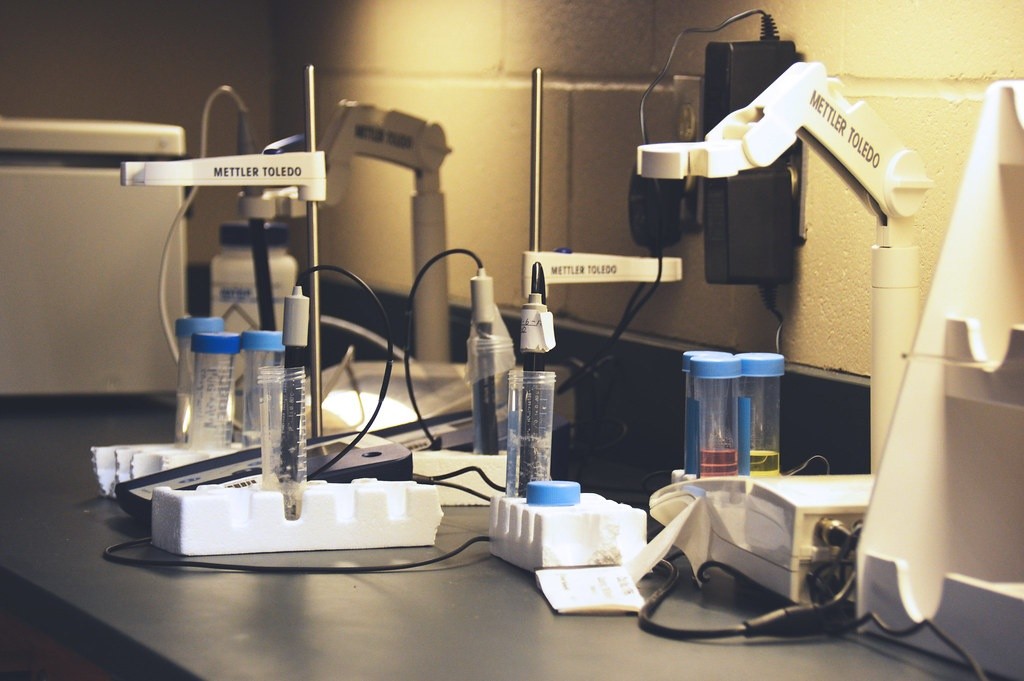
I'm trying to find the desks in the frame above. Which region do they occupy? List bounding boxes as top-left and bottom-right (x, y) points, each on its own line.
(0, 412), (994, 681)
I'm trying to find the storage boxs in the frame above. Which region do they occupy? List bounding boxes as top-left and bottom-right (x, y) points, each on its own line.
(0, 118), (189, 396)
